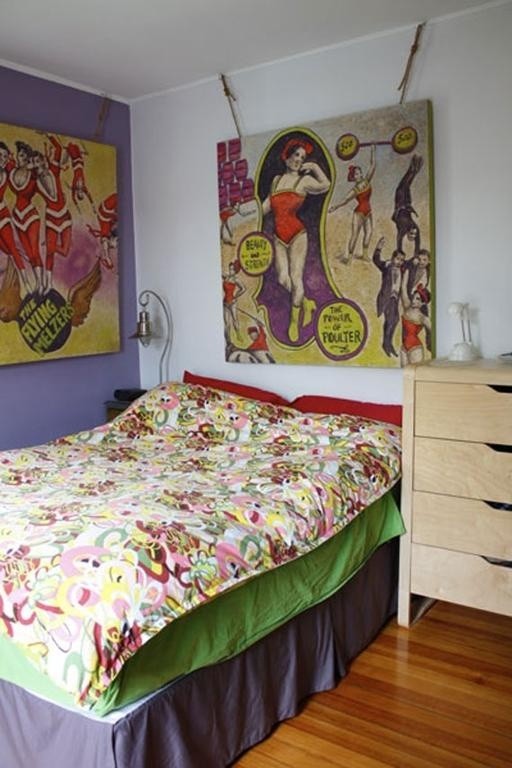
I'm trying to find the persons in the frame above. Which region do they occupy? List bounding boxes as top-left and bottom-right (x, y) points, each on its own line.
(1, 129), (120, 297)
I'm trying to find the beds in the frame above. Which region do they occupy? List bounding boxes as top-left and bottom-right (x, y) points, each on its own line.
(1, 381), (403, 767)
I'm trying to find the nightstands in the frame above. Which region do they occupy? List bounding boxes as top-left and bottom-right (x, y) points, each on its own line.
(104, 401), (131, 421)
(397, 357), (512, 629)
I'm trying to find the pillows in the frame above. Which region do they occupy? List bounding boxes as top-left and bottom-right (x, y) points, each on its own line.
(182, 371), (292, 406)
(290, 396), (403, 426)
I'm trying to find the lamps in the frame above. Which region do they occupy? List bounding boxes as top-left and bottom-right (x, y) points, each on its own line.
(447, 301), (483, 362)
(127, 290), (170, 384)
